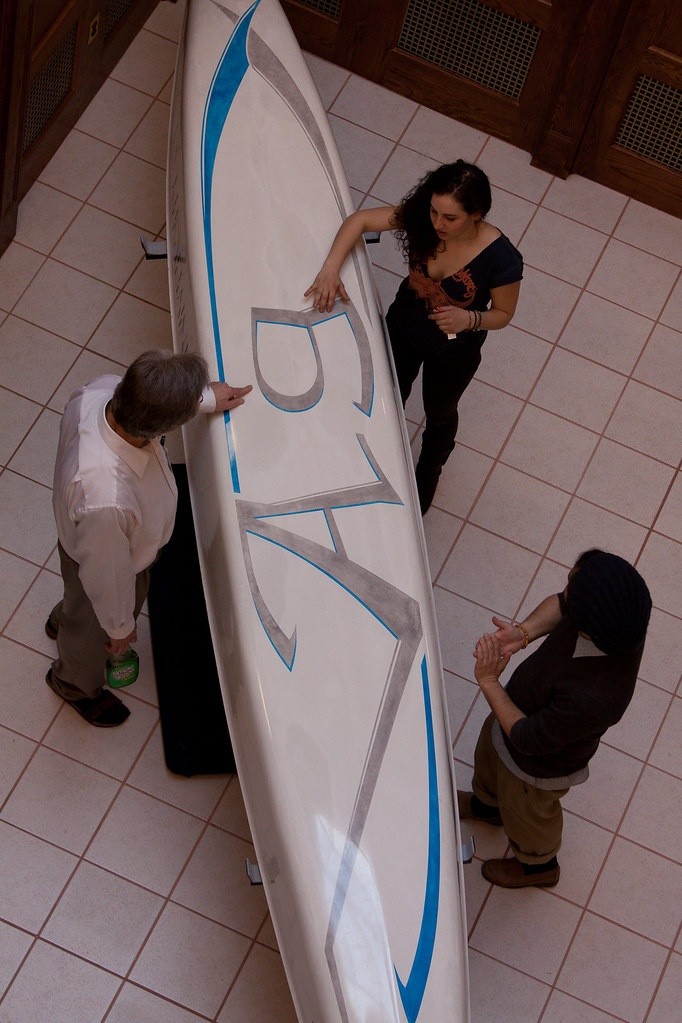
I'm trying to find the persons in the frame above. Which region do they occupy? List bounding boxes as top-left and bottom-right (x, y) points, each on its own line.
(456, 549), (652, 888)
(45, 350), (253, 727)
(304, 159), (524, 516)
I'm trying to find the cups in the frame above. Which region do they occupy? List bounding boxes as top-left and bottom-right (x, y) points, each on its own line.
(104, 650), (139, 689)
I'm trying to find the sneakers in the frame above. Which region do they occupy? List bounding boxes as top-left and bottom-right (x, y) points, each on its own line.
(457, 789), (504, 826)
(482, 856), (560, 888)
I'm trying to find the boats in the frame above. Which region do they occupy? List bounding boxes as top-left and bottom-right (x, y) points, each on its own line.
(170, 0), (476, 1023)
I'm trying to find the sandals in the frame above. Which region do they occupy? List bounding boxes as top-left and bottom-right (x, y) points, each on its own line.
(45, 618), (58, 639)
(46, 668), (130, 727)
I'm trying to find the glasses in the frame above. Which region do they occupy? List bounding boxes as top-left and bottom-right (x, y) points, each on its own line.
(197, 394), (203, 402)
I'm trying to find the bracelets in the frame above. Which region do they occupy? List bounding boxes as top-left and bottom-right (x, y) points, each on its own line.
(476, 311), (482, 329)
(516, 624), (528, 648)
(470, 311), (477, 330)
(466, 310), (471, 332)
